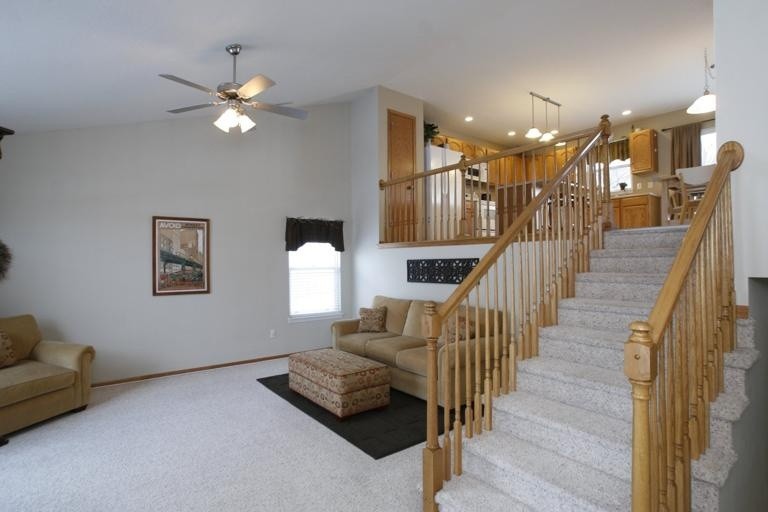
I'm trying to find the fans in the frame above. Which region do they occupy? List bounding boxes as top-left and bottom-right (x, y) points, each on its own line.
(158, 45), (313, 114)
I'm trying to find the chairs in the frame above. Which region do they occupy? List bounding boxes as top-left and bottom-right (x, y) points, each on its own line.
(1, 313), (95, 438)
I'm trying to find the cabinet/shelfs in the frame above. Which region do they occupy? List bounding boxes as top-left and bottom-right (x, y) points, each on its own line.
(433, 136), (525, 185)
(525, 147), (583, 182)
(604, 195), (662, 228)
(629, 128), (658, 175)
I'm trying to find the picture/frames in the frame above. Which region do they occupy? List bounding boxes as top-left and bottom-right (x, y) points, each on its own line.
(151, 215), (209, 295)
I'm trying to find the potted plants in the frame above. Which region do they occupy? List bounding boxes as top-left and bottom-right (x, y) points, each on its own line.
(424, 122), (439, 147)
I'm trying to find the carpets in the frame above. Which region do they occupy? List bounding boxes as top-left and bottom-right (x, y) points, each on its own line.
(257, 372), (484, 460)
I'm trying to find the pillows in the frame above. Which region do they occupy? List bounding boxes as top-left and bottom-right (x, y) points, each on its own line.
(436, 314), (479, 348)
(2, 332), (16, 367)
(357, 305), (387, 331)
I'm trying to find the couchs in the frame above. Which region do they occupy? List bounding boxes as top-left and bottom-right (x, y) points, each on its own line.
(331, 296), (510, 407)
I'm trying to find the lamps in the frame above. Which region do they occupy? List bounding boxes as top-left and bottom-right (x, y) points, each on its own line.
(685, 48), (717, 115)
(213, 108), (255, 132)
(524, 91), (567, 147)
